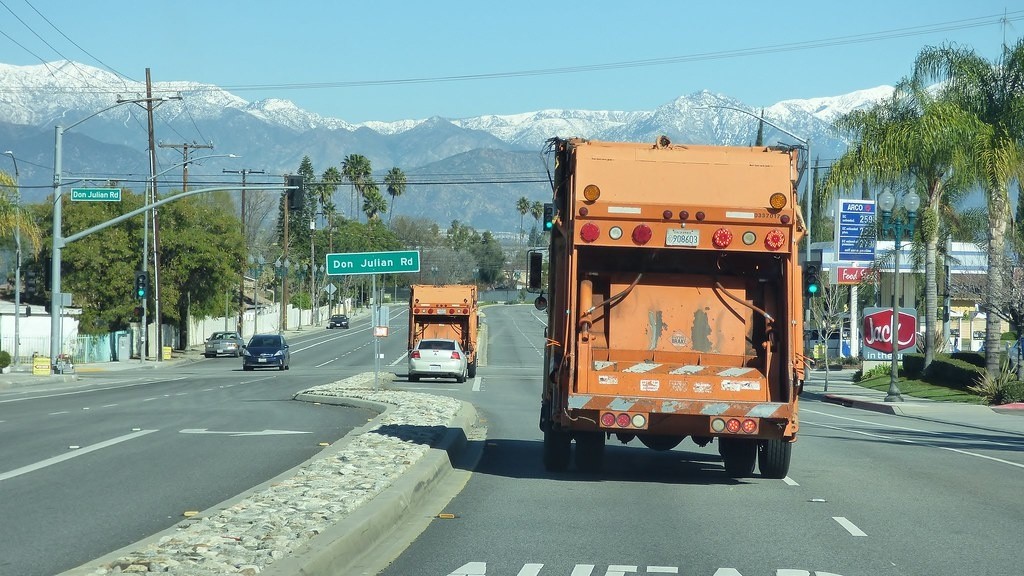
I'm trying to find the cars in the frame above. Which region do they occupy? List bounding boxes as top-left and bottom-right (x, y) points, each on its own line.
(205, 330), (244, 358)
(408, 338), (470, 384)
(328, 314), (350, 330)
(242, 334), (291, 371)
(1008, 336), (1024, 382)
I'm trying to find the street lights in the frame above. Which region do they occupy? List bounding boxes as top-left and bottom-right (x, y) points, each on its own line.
(249, 253), (267, 335)
(275, 257), (291, 334)
(140, 154), (243, 366)
(313, 264), (326, 328)
(293, 260), (310, 332)
(876, 183), (921, 402)
(50, 97), (172, 374)
(5, 150), (21, 367)
(690, 105), (811, 382)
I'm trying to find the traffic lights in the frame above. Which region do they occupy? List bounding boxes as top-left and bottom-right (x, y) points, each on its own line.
(543, 203), (553, 231)
(802, 260), (822, 297)
(134, 271), (150, 299)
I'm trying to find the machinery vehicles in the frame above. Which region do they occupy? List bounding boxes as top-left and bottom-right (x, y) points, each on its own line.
(407, 283), (481, 378)
(527, 133), (811, 478)
